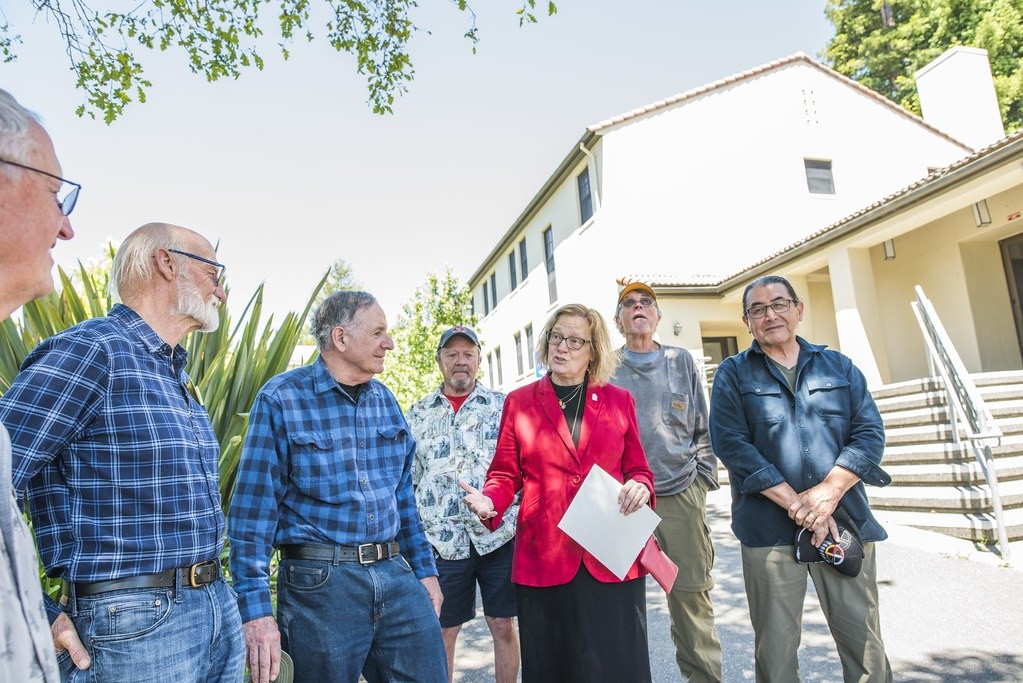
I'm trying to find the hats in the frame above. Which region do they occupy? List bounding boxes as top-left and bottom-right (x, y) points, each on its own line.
(795, 522), (865, 577)
(248, 650), (294, 683)
(436, 326), (481, 351)
(617, 283), (657, 301)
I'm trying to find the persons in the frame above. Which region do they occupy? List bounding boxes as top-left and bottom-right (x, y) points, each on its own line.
(406, 326), (520, 682)
(611, 283), (724, 683)
(709, 276), (893, 683)
(226, 290), (450, 683)
(0, 88), (76, 683)
(458, 304), (656, 682)
(0, 222), (245, 683)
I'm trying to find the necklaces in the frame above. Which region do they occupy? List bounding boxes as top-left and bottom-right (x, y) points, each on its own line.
(558, 382), (584, 437)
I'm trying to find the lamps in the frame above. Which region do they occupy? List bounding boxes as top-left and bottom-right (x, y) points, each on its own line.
(882, 238), (896, 261)
(972, 199), (992, 228)
(674, 323), (683, 336)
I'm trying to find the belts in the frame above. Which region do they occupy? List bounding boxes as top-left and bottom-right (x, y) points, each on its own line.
(280, 540), (399, 563)
(76, 559), (220, 597)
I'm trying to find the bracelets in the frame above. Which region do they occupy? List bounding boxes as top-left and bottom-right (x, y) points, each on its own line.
(479, 516), (487, 520)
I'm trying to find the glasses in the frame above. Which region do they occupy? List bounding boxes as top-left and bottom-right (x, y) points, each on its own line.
(545, 330), (593, 350)
(0, 159), (81, 216)
(152, 249), (226, 284)
(746, 299), (795, 319)
(617, 297), (655, 309)
